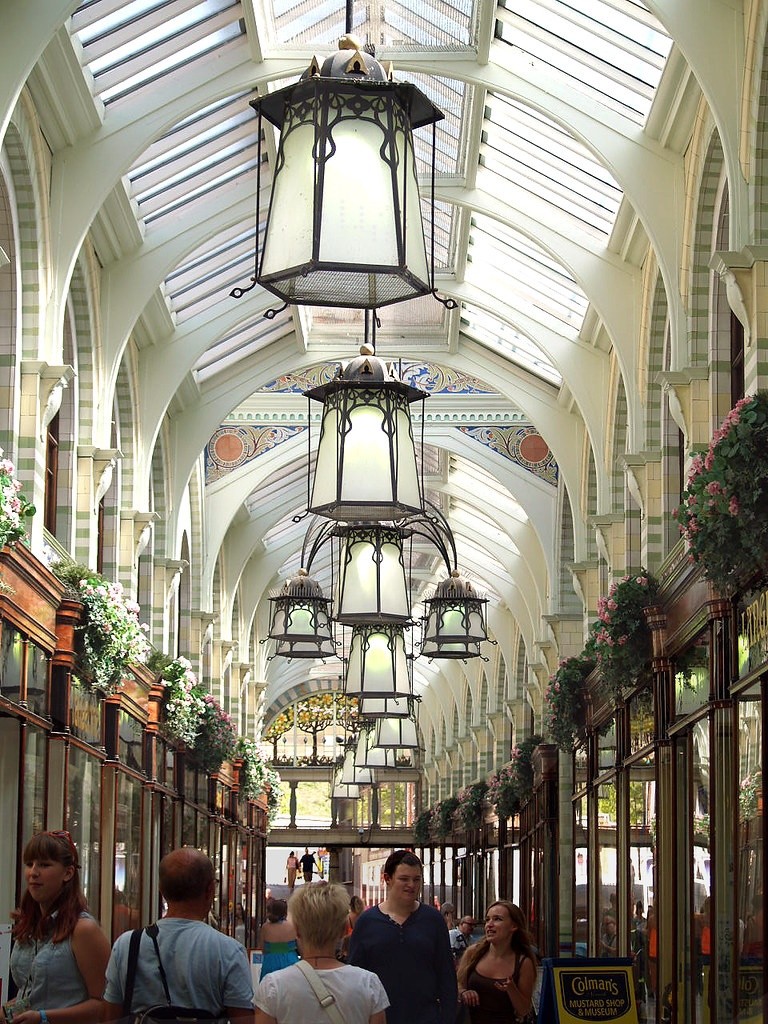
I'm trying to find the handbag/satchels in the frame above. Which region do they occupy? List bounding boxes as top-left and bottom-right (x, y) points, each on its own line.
(138, 1005), (218, 1024)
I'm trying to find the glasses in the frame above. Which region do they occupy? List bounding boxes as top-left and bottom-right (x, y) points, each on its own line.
(32, 831), (73, 851)
(463, 922), (476, 926)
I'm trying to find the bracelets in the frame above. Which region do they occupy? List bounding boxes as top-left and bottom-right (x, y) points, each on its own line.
(40, 1010), (47, 1023)
(460, 990), (468, 996)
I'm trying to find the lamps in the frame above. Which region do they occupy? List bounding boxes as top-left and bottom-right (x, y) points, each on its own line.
(228, 1), (499, 800)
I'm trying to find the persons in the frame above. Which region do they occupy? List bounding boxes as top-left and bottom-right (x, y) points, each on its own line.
(457, 901), (538, 1024)
(442, 901), (475, 949)
(0, 829), (111, 1024)
(299, 848), (320, 882)
(287, 851), (300, 891)
(266, 888), (275, 903)
(252, 880), (391, 1024)
(348, 849), (457, 1024)
(346, 896), (365, 931)
(260, 899), (298, 982)
(600, 893), (710, 997)
(105, 848), (254, 1024)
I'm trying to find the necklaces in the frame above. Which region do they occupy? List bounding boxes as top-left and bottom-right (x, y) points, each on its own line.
(306, 956), (334, 959)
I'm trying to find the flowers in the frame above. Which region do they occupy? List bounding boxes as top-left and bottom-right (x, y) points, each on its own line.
(0, 444), (279, 818)
(406, 389), (767, 842)
